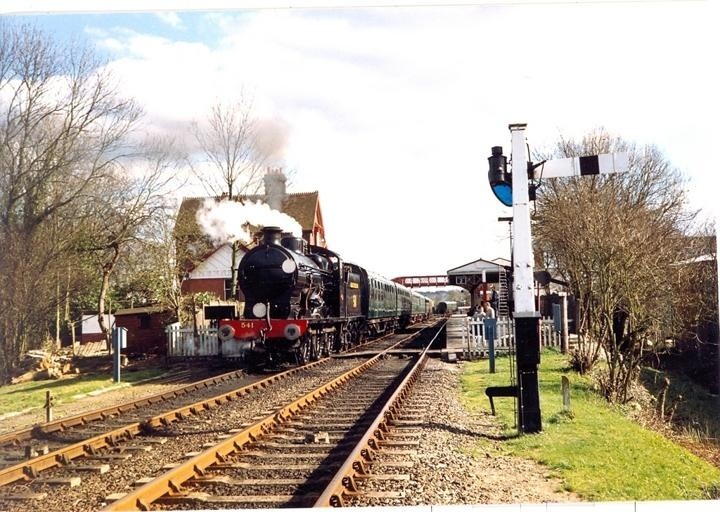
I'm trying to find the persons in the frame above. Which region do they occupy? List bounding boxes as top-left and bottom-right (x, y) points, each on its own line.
(473, 286), (499, 321)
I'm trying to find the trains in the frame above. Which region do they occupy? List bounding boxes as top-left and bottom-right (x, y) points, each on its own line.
(218, 226), (437, 366)
(439, 300), (461, 314)
